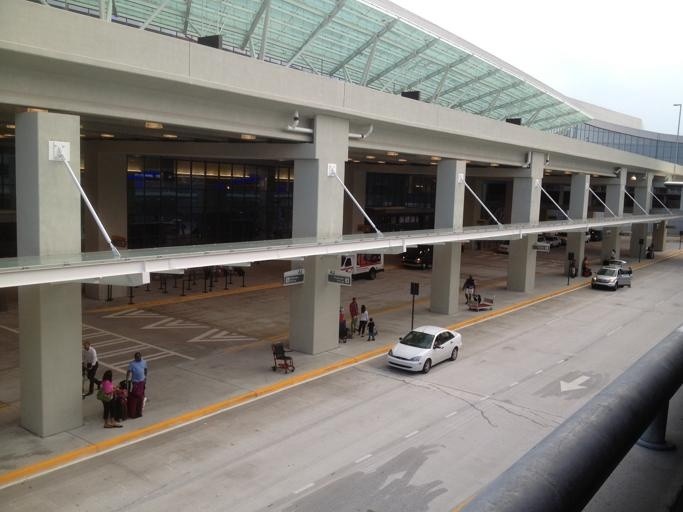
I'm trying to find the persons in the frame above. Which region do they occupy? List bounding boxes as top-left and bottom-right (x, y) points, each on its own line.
(102, 370), (123, 428)
(349, 297), (359, 335)
(583, 256), (591, 271)
(367, 318), (376, 341)
(462, 275), (476, 304)
(82, 342), (102, 396)
(124, 352), (148, 395)
(357, 304), (369, 337)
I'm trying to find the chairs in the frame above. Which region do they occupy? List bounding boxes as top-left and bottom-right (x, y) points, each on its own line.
(271, 340), (295, 374)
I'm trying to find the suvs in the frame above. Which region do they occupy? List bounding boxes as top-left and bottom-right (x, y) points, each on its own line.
(591, 259), (633, 292)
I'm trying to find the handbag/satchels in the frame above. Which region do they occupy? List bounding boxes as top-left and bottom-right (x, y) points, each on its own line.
(97, 391), (113, 401)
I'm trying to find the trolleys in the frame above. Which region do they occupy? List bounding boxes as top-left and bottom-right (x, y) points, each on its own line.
(466, 293), (496, 311)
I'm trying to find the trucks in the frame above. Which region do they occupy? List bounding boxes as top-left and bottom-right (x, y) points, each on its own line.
(339, 253), (385, 280)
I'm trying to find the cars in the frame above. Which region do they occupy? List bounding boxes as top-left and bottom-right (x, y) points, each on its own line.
(558, 232), (567, 245)
(401, 245), (433, 269)
(386, 325), (462, 374)
(591, 229), (602, 241)
(585, 231), (591, 243)
(546, 235), (562, 249)
(536, 235), (551, 254)
(498, 240), (510, 255)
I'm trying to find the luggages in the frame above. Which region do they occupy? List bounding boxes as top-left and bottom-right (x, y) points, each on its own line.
(126, 381), (141, 418)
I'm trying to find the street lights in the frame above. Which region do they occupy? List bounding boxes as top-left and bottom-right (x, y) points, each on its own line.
(672, 103), (682, 176)
